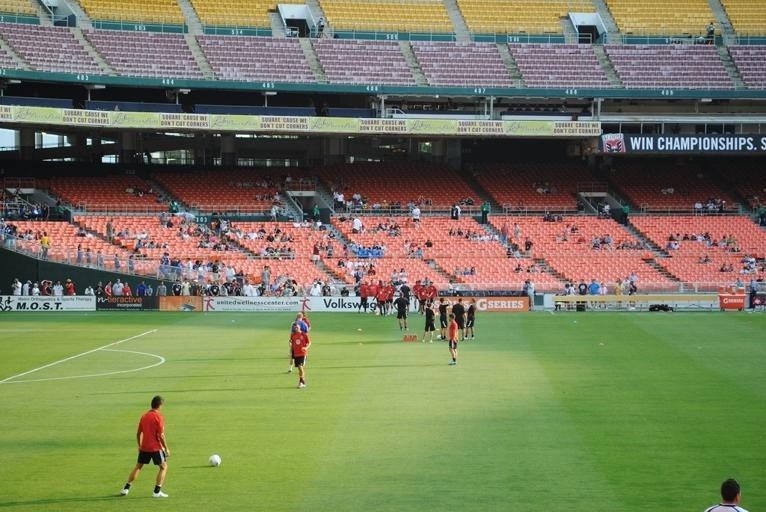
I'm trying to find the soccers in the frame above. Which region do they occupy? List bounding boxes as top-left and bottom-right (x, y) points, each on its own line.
(210, 455), (222, 467)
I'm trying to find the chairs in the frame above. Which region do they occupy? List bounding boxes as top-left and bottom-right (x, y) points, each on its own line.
(2, 0), (766, 93)
(2, 161), (763, 299)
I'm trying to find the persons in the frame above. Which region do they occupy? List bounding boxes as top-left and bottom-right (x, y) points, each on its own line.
(438, 297), (451, 341)
(704, 478), (749, 512)
(464, 297), (476, 340)
(422, 303), (436, 343)
(452, 299), (467, 341)
(120, 395), (170, 498)
(356, 282), (369, 314)
(286, 311), (311, 388)
(448, 313), (458, 365)
(373, 280), (390, 318)
(393, 292), (410, 332)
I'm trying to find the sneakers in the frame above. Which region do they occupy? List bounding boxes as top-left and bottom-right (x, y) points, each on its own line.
(120, 488), (128, 495)
(153, 491), (168, 497)
